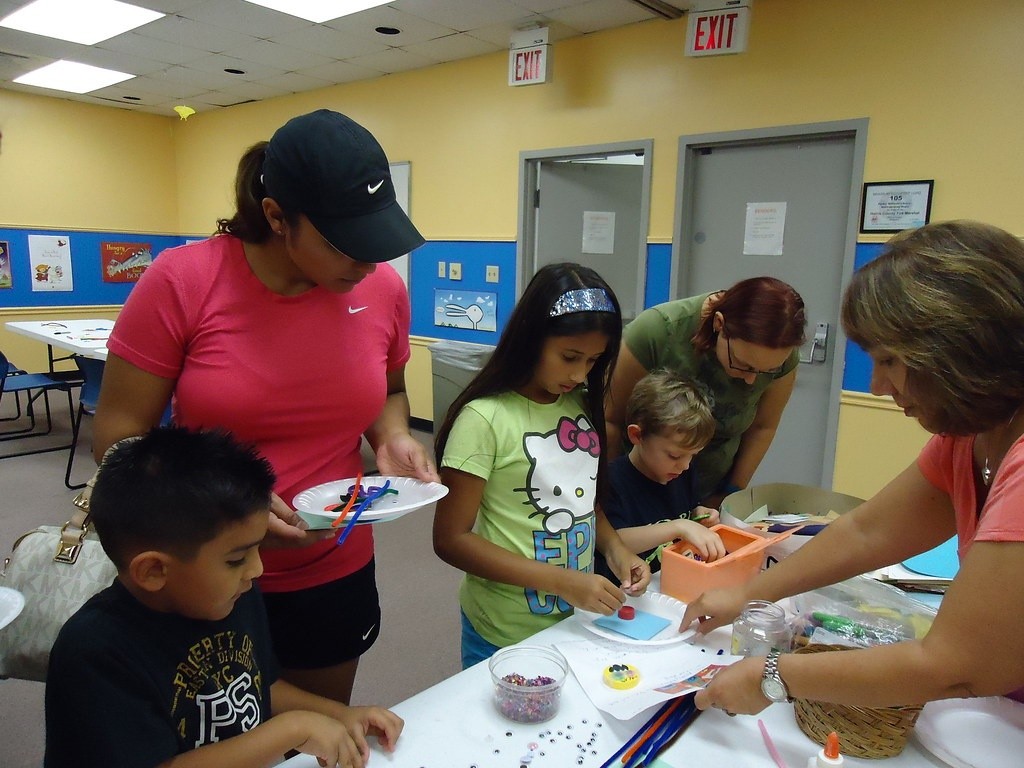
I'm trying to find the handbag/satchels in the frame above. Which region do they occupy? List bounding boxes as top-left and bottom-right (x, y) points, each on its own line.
(0, 432), (149, 680)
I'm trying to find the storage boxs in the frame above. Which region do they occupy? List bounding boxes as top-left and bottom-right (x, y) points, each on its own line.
(660, 523), (807, 606)
(721, 480), (868, 553)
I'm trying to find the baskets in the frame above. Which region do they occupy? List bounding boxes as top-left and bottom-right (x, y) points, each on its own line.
(790, 644), (924, 760)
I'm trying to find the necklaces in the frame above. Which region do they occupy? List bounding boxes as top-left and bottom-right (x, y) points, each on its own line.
(981, 405), (1020, 485)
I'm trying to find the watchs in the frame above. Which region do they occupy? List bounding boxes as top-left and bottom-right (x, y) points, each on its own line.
(759, 650), (796, 704)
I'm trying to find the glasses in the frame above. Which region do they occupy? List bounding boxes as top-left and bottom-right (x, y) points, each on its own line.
(725, 333), (786, 377)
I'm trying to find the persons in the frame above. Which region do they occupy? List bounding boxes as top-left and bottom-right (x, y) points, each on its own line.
(42, 423), (405, 768)
(92, 105), (444, 761)
(430, 262), (653, 671)
(602, 276), (808, 510)
(592, 367), (726, 590)
(679, 219), (1024, 716)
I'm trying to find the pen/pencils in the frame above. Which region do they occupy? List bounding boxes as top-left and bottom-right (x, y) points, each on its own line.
(54, 331), (71, 334)
(67, 336), (73, 339)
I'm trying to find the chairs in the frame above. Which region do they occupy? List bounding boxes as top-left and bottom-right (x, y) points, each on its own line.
(0, 351), (172, 490)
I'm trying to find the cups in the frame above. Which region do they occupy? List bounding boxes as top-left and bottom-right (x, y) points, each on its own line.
(489, 644), (568, 724)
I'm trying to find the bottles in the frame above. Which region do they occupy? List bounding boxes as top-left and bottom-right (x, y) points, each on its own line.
(730, 599), (792, 657)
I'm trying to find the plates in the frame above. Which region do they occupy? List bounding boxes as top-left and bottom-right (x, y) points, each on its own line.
(574, 590), (701, 645)
(292, 476), (449, 520)
(914, 696), (1024, 768)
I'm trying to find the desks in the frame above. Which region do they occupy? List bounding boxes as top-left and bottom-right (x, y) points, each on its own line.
(6, 318), (116, 360)
(272, 510), (1024, 768)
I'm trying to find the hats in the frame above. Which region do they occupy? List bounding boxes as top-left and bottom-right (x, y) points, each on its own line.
(265, 109), (426, 265)
